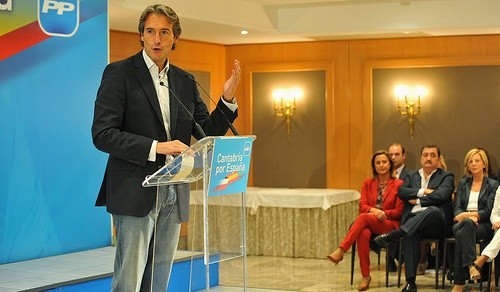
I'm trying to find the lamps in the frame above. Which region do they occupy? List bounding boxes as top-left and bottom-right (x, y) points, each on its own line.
(271, 88), (302, 137)
(394, 85), (427, 140)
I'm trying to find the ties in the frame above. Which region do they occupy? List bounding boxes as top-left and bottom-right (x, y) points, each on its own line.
(392, 169), (396, 178)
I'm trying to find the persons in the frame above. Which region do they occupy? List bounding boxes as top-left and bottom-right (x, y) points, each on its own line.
(92, 4), (242, 292)
(327, 142), (500, 292)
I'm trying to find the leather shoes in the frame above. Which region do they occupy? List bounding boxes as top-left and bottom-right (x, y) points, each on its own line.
(401, 282), (417, 292)
(375, 233), (394, 247)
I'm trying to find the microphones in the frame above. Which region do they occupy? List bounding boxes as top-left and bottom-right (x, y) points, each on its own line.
(160, 82), (206, 137)
(185, 73), (239, 136)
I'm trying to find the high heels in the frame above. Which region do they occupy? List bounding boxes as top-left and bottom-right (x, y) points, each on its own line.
(469, 266), (481, 284)
(326, 255), (343, 267)
(358, 276), (371, 292)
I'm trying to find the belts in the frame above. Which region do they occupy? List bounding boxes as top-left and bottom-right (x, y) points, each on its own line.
(162, 167), (177, 174)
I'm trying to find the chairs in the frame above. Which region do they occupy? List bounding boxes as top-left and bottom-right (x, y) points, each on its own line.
(350, 236), (500, 292)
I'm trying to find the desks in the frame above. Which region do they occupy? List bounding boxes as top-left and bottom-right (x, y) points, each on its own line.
(186, 187), (361, 259)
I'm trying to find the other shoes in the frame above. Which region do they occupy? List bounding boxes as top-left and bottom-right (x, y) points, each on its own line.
(431, 246), (439, 256)
(387, 256), (396, 272)
(417, 261), (428, 275)
(473, 262), (482, 270)
(447, 270), (455, 279)
